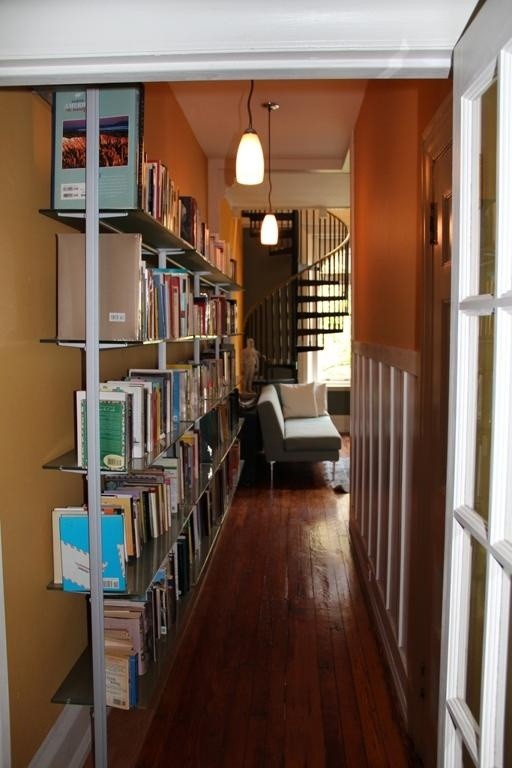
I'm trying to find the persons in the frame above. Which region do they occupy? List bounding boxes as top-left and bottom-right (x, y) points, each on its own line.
(243, 338), (259, 394)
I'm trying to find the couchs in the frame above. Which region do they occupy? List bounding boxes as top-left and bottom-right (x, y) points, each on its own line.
(257, 382), (342, 490)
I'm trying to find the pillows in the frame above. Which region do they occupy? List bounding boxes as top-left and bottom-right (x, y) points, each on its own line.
(317, 383), (330, 416)
(279, 382), (319, 417)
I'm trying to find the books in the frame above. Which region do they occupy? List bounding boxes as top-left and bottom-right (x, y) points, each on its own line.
(55, 87), (238, 282)
(54, 390), (244, 708)
(57, 231), (236, 342)
(73, 343), (238, 472)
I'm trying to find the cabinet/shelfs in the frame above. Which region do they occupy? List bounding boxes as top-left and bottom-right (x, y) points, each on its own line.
(39, 82), (244, 768)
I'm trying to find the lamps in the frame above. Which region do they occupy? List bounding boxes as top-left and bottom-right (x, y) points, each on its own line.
(260, 100), (279, 246)
(235, 80), (264, 186)
